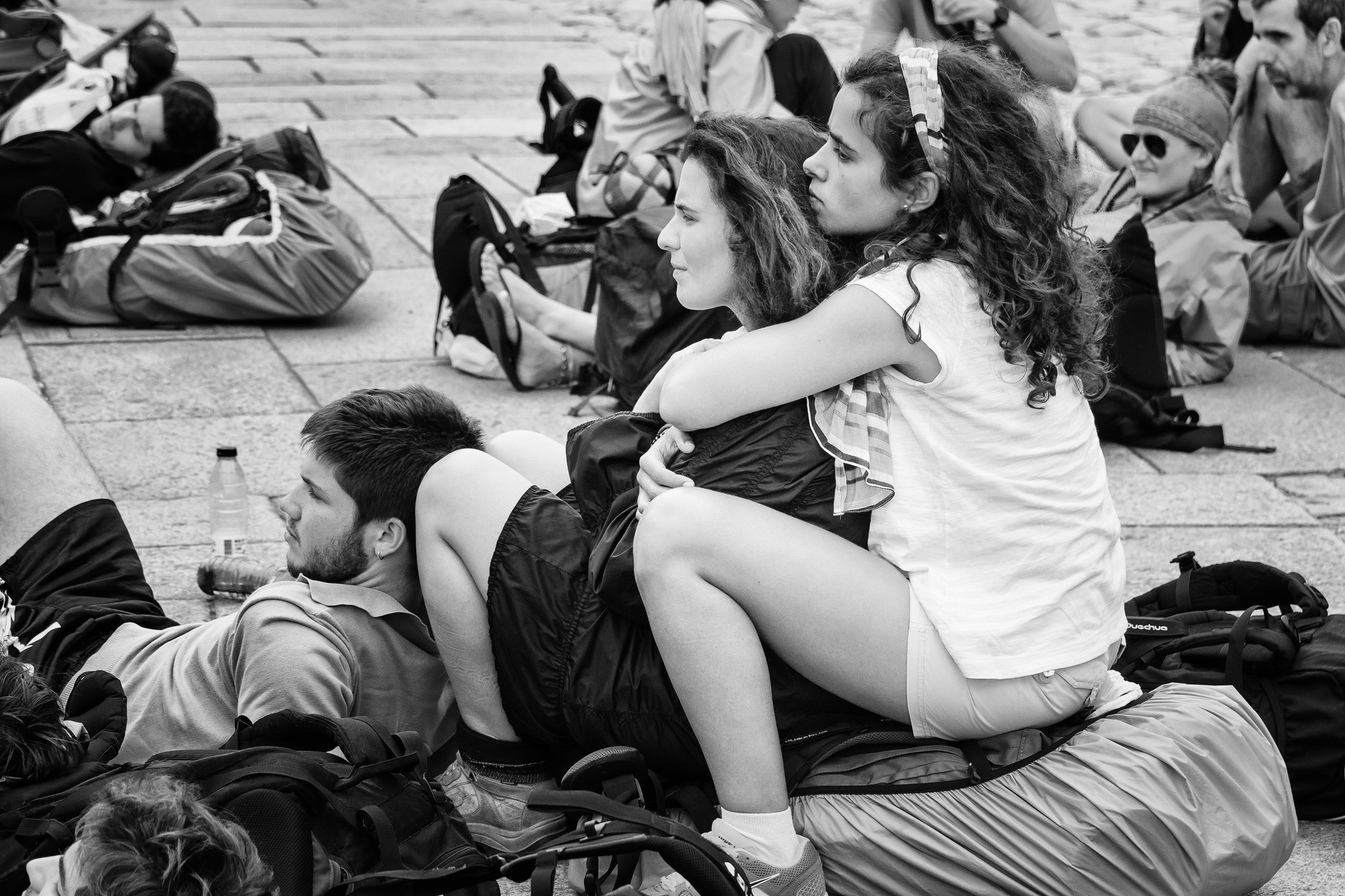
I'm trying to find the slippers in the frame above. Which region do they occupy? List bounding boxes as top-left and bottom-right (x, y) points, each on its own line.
(469, 235), (536, 392)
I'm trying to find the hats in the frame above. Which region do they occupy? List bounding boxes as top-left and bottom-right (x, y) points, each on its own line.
(1132, 55), (1234, 159)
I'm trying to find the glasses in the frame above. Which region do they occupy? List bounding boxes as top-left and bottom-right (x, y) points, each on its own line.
(1119, 133), (1171, 161)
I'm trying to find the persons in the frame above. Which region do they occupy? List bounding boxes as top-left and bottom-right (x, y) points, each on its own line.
(1, 0), (1345, 896)
(633, 44), (1128, 896)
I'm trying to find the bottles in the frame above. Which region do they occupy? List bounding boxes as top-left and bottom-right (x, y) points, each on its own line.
(208, 445), (249, 557)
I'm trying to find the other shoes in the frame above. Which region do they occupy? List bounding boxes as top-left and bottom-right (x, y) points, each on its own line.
(640, 818), (829, 896)
(435, 748), (565, 853)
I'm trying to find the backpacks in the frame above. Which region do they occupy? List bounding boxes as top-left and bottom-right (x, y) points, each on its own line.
(0, 706), (500, 896)
(1084, 213), (1276, 453)
(565, 204), (744, 422)
(513, 65), (604, 215)
(1111, 550), (1345, 822)
(432, 172), (550, 359)
